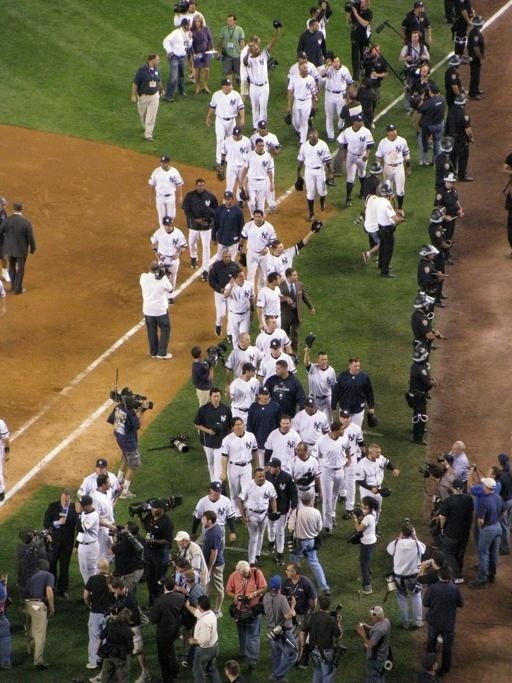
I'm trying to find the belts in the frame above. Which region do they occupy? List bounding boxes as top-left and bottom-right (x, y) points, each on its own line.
(324, 88), (353, 92)
(229, 460), (253, 467)
(162, 254), (175, 258)
(247, 507), (267, 515)
(393, 571), (422, 581)
(294, 97), (311, 100)
(255, 251), (260, 253)
(253, 248), (264, 251)
(251, 177), (263, 180)
(296, 484), (317, 491)
(158, 193), (172, 198)
(302, 441), (317, 447)
(229, 310), (251, 314)
(386, 163), (404, 167)
(264, 314), (280, 321)
(215, 113), (237, 120)
(297, 537), (315, 544)
(312, 166), (325, 171)
(309, 393), (329, 401)
(248, 79), (268, 87)
(76, 539), (98, 546)
(347, 150), (366, 157)
(233, 406), (251, 413)
(321, 465), (343, 473)
(26, 597), (42, 602)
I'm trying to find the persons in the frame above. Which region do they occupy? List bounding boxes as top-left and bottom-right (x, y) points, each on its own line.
(287, 490), (329, 595)
(74, 496), (99, 584)
(263, 575), (300, 682)
(297, 129), (333, 222)
(219, 418), (260, 502)
(405, 348), (437, 445)
(419, 85), (446, 166)
(361, 162), (382, 200)
(469, 478), (505, 586)
(467, 15), (486, 100)
(1, 202), (36, 293)
(0, 280), (6, 317)
(107, 388), (142, 497)
(362, 44), (387, 100)
(345, 0), (373, 78)
(189, 16), (216, 95)
(223, 363), (259, 430)
(211, 192), (244, 264)
(42, 494), (78, 599)
(133, 55), (167, 140)
(20, 559), (55, 669)
(223, 332), (263, 383)
(142, 498), (173, 593)
(434, 137), (456, 191)
(149, 216), (188, 305)
(162, 18), (195, 103)
(175, 532), (211, 588)
(99, 608), (133, 683)
(339, 86), (365, 126)
(303, 332), (337, 425)
(310, 423), (350, 535)
(174, 559), (200, 593)
(288, 51), (319, 88)
(222, 661), (244, 682)
(306, 1), (329, 42)
(444, 56), (468, 106)
(264, 231), (315, 283)
(410, 291), (444, 360)
(503, 151), (512, 254)
(466, 464), (502, 573)
(296, 20), (327, 69)
(331, 358), (376, 429)
(216, 14), (246, 83)
(279, 269), (316, 353)
(1, 196), (11, 282)
(353, 497), (379, 597)
(84, 558), (115, 670)
(263, 459), (298, 563)
(357, 607), (392, 681)
(264, 415), (303, 473)
(74, 459), (121, 505)
(336, 115), (373, 206)
(361, 183), (389, 266)
(200, 511), (226, 619)
(299, 593), (344, 682)
(107, 523), (150, 628)
(374, 181), (404, 279)
(191, 346), (221, 408)
(438, 442), (471, 500)
(282, 563), (317, 665)
(243, 29), (279, 130)
(1, 420), (10, 501)
(209, 250), (240, 336)
(388, 521), (426, 627)
(246, 387), (283, 450)
(240, 139), (274, 218)
(291, 399), (331, 447)
(225, 561), (268, 666)
(174, 2), (204, 29)
(354, 443), (399, 538)
(374, 125), (412, 208)
(446, 94), (476, 182)
(405, 64), (435, 131)
(421, 568), (465, 675)
(416, 244), (444, 298)
(259, 340), (297, 384)
(183, 597), (222, 682)
(189, 481), (239, 547)
(222, 268), (255, 348)
(429, 208), (450, 267)
(284, 441), (321, 510)
(255, 315), (293, 354)
(254, 271), (292, 331)
(287, 64), (317, 142)
(219, 126), (252, 199)
(240, 36), (262, 101)
(87, 475), (118, 563)
(263, 362), (308, 415)
(90, 577), (151, 682)
(150, 576), (187, 682)
(206, 78), (244, 180)
(146, 156), (185, 231)
(439, 480), (473, 585)
(317, 55), (357, 143)
(138, 261), (174, 361)
(176, 571), (204, 618)
(358, 79), (375, 126)
(401, 2), (433, 51)
(399, 30), (430, 117)
(336, 408), (365, 518)
(235, 470), (279, 568)
(452, 0), (474, 63)
(419, 552), (444, 585)
(183, 178), (218, 282)
(444, 1), (457, 25)
(192, 387), (234, 482)
(496, 453), (512, 556)
(432, 172), (464, 245)
(249, 120), (281, 204)
(238, 210), (278, 289)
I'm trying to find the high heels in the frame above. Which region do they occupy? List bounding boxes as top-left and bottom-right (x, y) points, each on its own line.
(195, 86), (201, 94)
(203, 85), (212, 93)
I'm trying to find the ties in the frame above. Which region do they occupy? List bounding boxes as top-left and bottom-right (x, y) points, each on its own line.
(179, 574), (184, 583)
(290, 282), (296, 302)
(61, 509), (66, 513)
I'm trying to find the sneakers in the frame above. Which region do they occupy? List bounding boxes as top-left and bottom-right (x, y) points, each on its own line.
(345, 198), (350, 207)
(332, 516), (335, 525)
(238, 652), (242, 661)
(359, 193), (364, 197)
(267, 542), (275, 552)
(227, 334), (233, 344)
(357, 576), (364, 582)
(182, 661), (188, 666)
(26, 648), (28, 650)
(339, 496), (344, 500)
(413, 619), (422, 625)
(276, 553), (284, 565)
(86, 662), (98, 666)
(378, 264), (392, 269)
(0, 491), (6, 497)
(145, 134), (150, 140)
(119, 491), (133, 497)
(381, 272), (395, 278)
(455, 577), (463, 581)
(249, 657), (256, 664)
(310, 213), (315, 219)
(362, 250), (372, 262)
(215, 325), (222, 336)
(151, 356), (155, 358)
(32, 661), (52, 666)
(474, 561), (481, 569)
(202, 271), (206, 281)
(376, 257), (380, 260)
(344, 511), (351, 518)
(325, 526), (330, 534)
(165, 97), (174, 102)
(0, 268), (12, 284)
(12, 288), (26, 291)
(178, 88), (184, 92)
(357, 588), (373, 598)
(470, 582), (482, 587)
(287, 539), (294, 549)
(191, 259), (196, 268)
(320, 203), (323, 209)
(134, 673), (151, 680)
(157, 353), (173, 359)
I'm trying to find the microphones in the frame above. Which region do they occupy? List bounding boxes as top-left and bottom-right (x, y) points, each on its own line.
(375, 18), (389, 34)
(58, 508), (68, 523)
(370, 484), (393, 500)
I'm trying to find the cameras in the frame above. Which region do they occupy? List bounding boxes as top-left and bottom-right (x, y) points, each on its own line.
(418, 461), (435, 478)
(174, 0), (190, 14)
(342, 509), (363, 520)
(202, 343), (227, 370)
(115, 529), (145, 553)
(157, 573), (175, 585)
(353, 212), (365, 225)
(436, 452), (454, 464)
(235, 596), (264, 625)
(383, 657), (393, 671)
(128, 495), (184, 519)
(343, 0), (362, 13)
(153, 263), (173, 280)
(384, 571), (398, 593)
(358, 45), (378, 79)
(166, 550), (180, 567)
(330, 644), (347, 668)
(267, 56), (279, 70)
(329, 603), (343, 617)
(466, 463), (479, 471)
(284, 585), (300, 601)
(298, 644), (310, 669)
(109, 367), (153, 413)
(408, 580), (423, 595)
(266, 624), (300, 654)
(406, 65), (421, 77)
(284, 535), (297, 554)
(170, 438), (190, 453)
(28, 528), (53, 550)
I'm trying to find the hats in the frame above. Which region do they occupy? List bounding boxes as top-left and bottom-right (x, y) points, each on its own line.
(386, 123), (397, 130)
(96, 459), (108, 468)
(159, 156), (170, 164)
(210, 481), (222, 493)
(285, 113), (292, 126)
(298, 51), (307, 57)
(352, 113), (361, 118)
(258, 120), (266, 125)
(224, 190), (232, 200)
(266, 457), (283, 467)
(296, 178), (304, 192)
(327, 177), (336, 187)
(498, 452), (508, 463)
(311, 220), (323, 235)
(480, 476), (497, 493)
(420, 653), (437, 666)
(415, 0), (424, 4)
(428, 81), (440, 91)
(378, 487), (392, 498)
(19, 528), (35, 544)
(232, 126), (242, 135)
(268, 510), (282, 521)
(270, 339), (280, 349)
(163, 217), (174, 226)
(268, 575), (283, 590)
(174, 531), (191, 543)
(364, 76), (375, 88)
(221, 78), (233, 83)
(306, 333), (315, 349)
(121, 387), (133, 396)
(150, 500), (166, 507)
(452, 479), (464, 490)
(430, 549), (447, 566)
(77, 494), (93, 509)
(258, 386), (270, 395)
(273, 20), (280, 25)
(239, 187), (248, 203)
(367, 412), (378, 427)
(340, 410), (351, 418)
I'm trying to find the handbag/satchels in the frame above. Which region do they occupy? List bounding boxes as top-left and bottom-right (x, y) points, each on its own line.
(390, 647), (394, 661)
(97, 621), (109, 656)
(137, 604), (150, 624)
(303, 611), (317, 650)
(353, 513), (373, 545)
(254, 567), (268, 609)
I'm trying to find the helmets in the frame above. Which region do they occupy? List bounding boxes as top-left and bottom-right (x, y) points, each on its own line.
(443, 170), (456, 183)
(448, 54), (459, 61)
(430, 208), (446, 224)
(413, 291), (432, 308)
(379, 178), (394, 196)
(470, 15), (483, 27)
(370, 162), (383, 175)
(420, 243), (441, 258)
(454, 92), (469, 104)
(441, 136), (455, 149)
(411, 345), (427, 362)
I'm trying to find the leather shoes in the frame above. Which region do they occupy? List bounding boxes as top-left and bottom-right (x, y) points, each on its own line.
(431, 343), (437, 348)
(60, 589), (68, 597)
(413, 437), (426, 445)
(439, 290), (447, 295)
(426, 393), (430, 397)
(424, 427), (427, 432)
(444, 259), (452, 262)
(460, 175), (473, 181)
(475, 89), (481, 91)
